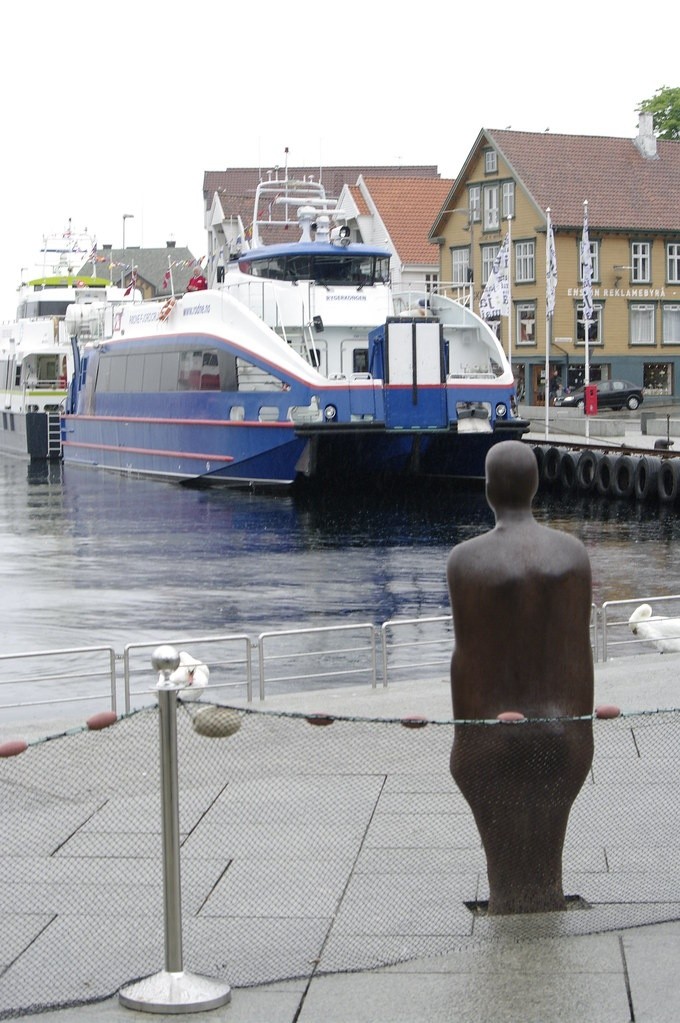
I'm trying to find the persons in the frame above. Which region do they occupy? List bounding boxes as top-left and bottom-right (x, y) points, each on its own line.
(550, 371), (560, 402)
(186, 265), (207, 291)
(446, 440), (595, 913)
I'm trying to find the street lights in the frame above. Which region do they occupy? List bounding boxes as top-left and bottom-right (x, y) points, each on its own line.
(122, 214), (133, 249)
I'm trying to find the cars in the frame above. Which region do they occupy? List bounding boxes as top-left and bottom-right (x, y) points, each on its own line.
(553, 381), (644, 411)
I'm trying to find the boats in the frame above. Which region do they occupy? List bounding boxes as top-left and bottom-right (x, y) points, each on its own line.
(0, 214), (142, 465)
(67, 148), (531, 494)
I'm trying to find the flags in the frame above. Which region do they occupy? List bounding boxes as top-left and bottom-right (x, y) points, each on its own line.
(162, 270), (170, 288)
(582, 216), (593, 319)
(479, 233), (509, 318)
(124, 271), (136, 295)
(545, 221), (558, 315)
